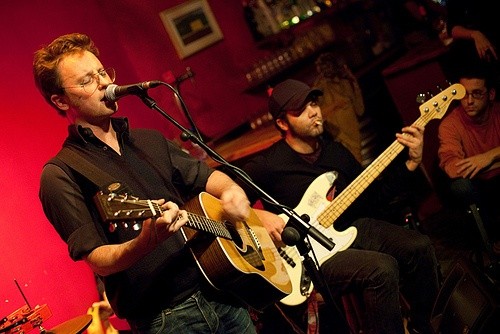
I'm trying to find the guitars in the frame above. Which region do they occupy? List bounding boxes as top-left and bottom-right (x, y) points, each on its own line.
(277, 79), (467, 307)
(96, 183), (293, 312)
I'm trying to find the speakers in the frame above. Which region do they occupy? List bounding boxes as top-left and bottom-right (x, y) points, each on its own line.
(426, 257), (500, 334)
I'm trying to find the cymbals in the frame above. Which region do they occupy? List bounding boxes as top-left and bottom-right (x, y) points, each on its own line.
(43, 314), (93, 334)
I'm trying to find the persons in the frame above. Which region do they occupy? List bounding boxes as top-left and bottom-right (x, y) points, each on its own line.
(32, 33), (257, 334)
(440, 0), (500, 81)
(235, 79), (444, 334)
(437, 66), (500, 225)
(325, 40), (407, 177)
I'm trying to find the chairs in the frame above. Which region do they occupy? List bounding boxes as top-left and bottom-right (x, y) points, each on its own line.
(342, 102), (493, 334)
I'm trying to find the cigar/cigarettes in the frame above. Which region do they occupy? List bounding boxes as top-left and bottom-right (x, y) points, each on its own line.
(314, 121), (323, 126)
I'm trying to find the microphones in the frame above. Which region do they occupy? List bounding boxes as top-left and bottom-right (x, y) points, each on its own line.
(104, 80), (162, 103)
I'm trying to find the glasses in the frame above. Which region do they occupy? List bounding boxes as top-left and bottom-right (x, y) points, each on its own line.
(59, 68), (117, 95)
(462, 90), (489, 99)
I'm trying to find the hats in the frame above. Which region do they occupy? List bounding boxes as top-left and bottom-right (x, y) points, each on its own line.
(268, 79), (324, 121)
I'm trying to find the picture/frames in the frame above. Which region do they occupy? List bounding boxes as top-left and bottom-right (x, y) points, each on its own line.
(159, 0), (224, 60)
